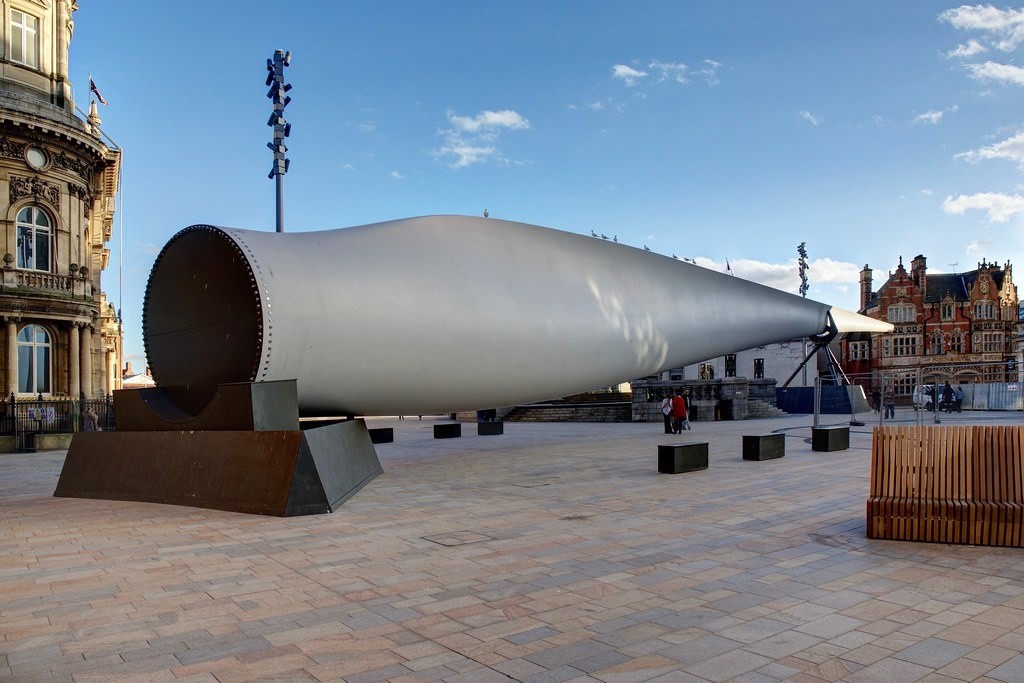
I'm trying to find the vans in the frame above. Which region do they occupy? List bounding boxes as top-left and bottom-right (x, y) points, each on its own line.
(913, 383), (957, 411)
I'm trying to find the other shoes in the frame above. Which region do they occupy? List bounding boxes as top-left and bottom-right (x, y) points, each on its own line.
(672, 430), (677, 434)
(679, 430), (681, 434)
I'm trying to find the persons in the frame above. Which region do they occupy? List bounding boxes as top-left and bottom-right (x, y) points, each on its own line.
(942, 384), (955, 413)
(954, 386), (965, 413)
(681, 390), (692, 431)
(660, 392), (675, 435)
(882, 384), (896, 419)
(928, 384), (940, 414)
(871, 388), (881, 415)
(673, 392), (687, 435)
